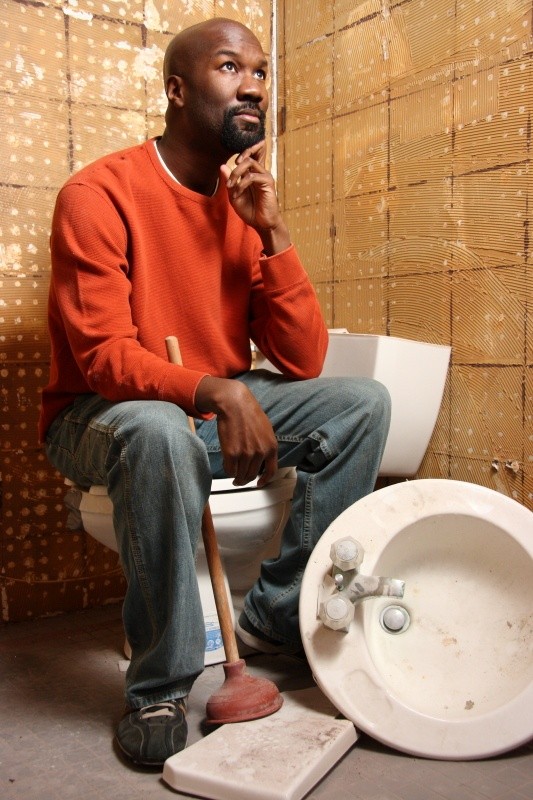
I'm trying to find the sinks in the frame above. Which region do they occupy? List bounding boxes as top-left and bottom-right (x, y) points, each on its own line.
(299, 478), (531, 759)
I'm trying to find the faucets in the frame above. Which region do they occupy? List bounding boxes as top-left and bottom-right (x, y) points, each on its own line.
(316, 535), (405, 632)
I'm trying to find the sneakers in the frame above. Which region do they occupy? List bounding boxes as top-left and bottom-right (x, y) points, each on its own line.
(114, 695), (189, 769)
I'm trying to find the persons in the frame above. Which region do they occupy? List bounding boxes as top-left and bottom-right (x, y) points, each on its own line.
(32, 17), (389, 769)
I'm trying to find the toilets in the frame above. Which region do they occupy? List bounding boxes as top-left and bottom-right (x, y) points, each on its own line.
(75, 326), (457, 670)
